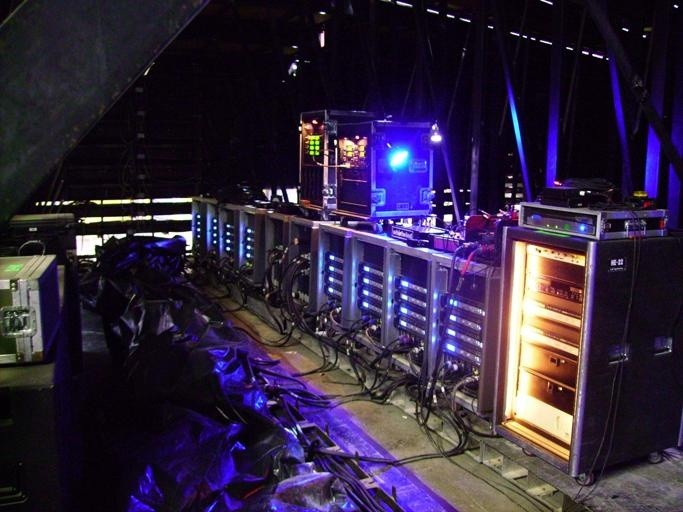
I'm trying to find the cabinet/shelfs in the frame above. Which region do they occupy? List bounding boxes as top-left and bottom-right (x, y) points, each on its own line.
(492, 226), (683, 484)
